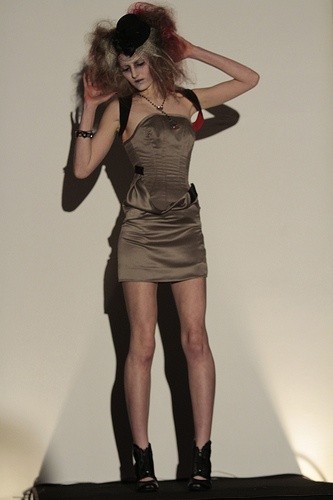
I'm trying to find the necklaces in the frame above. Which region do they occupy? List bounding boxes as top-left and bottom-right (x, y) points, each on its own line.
(136, 89), (177, 128)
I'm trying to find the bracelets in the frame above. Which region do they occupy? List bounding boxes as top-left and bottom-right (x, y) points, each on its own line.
(75, 129), (96, 138)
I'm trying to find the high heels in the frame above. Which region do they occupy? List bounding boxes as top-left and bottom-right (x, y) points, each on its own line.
(131, 442), (159, 493)
(186, 441), (211, 490)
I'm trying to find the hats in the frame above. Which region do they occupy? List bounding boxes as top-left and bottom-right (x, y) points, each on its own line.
(113, 14), (151, 56)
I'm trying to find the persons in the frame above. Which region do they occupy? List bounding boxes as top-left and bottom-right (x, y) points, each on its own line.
(73, 2), (260, 491)
